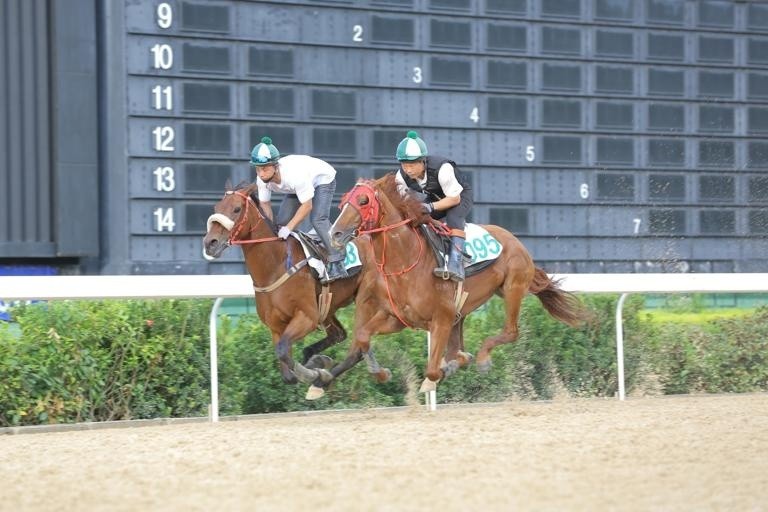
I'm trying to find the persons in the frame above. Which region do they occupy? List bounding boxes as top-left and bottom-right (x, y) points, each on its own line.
(248, 136), (347, 285)
(394, 130), (475, 283)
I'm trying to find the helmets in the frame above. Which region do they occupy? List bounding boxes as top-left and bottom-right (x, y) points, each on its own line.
(249, 137), (281, 166)
(396, 131), (428, 161)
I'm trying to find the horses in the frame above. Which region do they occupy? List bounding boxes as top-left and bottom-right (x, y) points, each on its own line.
(203, 177), (407, 401)
(327, 172), (597, 394)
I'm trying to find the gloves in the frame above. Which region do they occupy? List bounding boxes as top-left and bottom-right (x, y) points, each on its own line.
(278, 226), (290, 241)
(420, 202), (435, 215)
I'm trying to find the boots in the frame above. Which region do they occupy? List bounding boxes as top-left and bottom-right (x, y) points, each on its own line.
(433, 229), (465, 283)
(320, 260), (349, 285)
